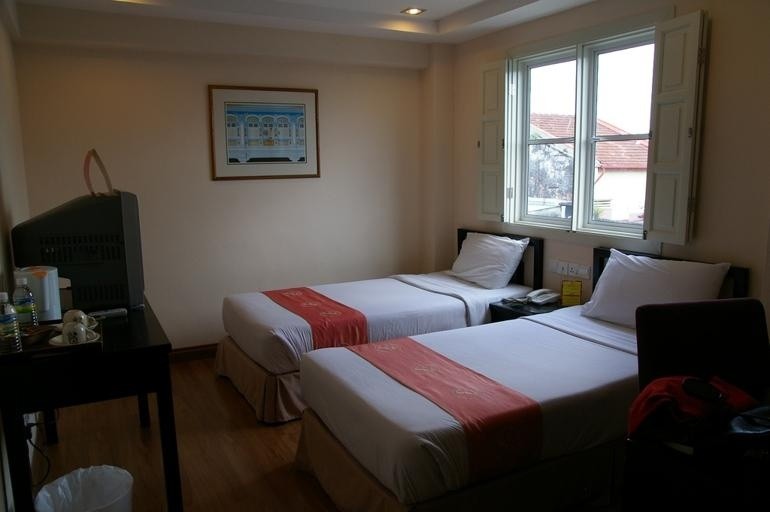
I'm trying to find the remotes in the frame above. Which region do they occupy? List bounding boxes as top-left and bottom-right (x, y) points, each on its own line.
(90, 308), (127, 319)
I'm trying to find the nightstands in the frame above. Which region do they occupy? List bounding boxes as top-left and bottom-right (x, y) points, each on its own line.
(488, 290), (582, 323)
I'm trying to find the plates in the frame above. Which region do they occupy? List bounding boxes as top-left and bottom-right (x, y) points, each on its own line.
(56, 323), (99, 330)
(48, 331), (101, 347)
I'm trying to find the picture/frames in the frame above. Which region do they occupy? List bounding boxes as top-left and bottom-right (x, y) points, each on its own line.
(207, 85), (321, 181)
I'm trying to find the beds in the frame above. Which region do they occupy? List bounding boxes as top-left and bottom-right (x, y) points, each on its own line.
(208, 227), (547, 422)
(292, 247), (752, 509)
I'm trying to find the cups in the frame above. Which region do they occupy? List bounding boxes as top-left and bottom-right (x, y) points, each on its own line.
(63, 309), (96, 326)
(62, 321), (95, 344)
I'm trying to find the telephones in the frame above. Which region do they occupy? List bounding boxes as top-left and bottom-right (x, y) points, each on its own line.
(526, 289), (561, 305)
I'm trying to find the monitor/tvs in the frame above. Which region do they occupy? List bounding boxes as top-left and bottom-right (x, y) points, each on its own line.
(11, 188), (145, 311)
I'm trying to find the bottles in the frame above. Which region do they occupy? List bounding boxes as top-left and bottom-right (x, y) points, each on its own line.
(13, 276), (39, 337)
(0, 293), (23, 356)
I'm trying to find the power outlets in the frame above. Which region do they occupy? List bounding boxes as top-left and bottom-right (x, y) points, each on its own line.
(568, 262), (578, 276)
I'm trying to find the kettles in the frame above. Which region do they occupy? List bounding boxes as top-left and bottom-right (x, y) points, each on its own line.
(13, 265), (62, 325)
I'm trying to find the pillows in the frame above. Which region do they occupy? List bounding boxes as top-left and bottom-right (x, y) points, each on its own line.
(449, 232), (534, 292)
(580, 248), (730, 326)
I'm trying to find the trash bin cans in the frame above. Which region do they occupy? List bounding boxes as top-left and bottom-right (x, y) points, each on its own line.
(34, 464), (134, 512)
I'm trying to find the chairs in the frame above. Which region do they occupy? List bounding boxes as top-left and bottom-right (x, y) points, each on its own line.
(632, 298), (770, 509)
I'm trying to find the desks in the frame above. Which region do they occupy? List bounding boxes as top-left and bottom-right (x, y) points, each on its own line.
(0, 289), (186, 512)
